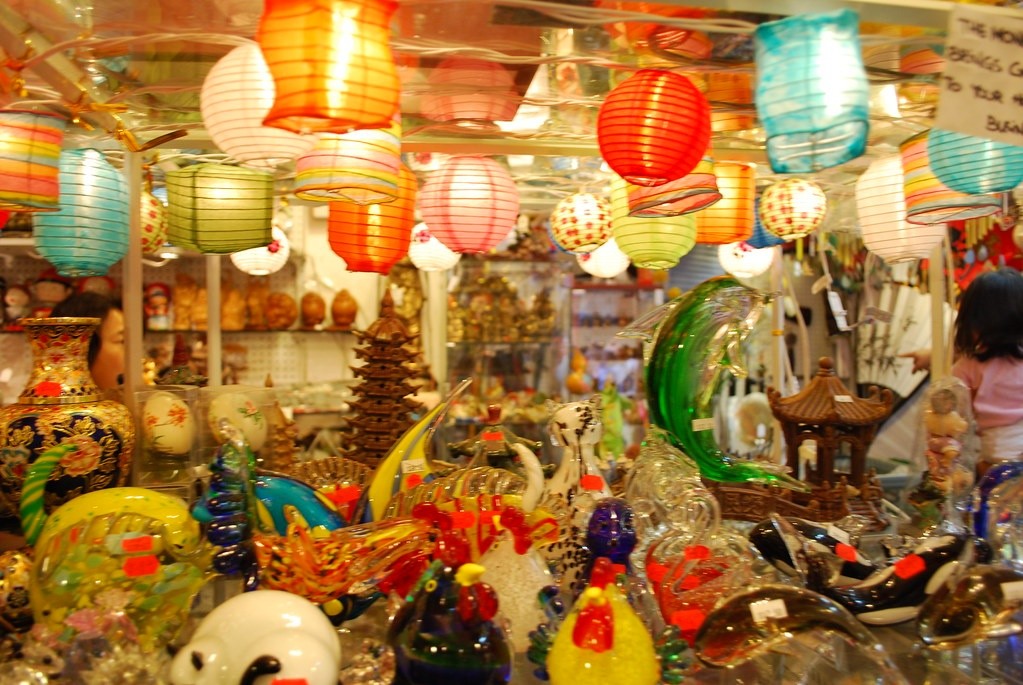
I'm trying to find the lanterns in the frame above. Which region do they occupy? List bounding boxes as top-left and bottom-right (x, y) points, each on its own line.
(0, 0), (1023, 305)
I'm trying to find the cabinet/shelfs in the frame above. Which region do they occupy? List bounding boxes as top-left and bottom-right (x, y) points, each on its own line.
(0, 233), (655, 457)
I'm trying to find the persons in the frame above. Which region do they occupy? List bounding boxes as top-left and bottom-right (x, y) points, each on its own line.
(0, 291), (125, 551)
(897, 268), (1023, 479)
